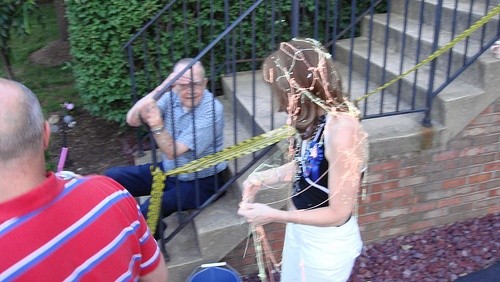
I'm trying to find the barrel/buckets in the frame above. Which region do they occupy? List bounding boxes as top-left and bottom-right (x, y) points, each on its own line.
(184, 262), (244, 282)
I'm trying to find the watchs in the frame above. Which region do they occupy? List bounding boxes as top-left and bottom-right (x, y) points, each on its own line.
(150, 125), (164, 135)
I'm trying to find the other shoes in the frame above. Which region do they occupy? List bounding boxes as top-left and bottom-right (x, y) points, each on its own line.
(153, 221), (167, 240)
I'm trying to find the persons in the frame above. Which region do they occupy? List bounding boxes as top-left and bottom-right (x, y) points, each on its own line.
(237, 36), (369, 282)
(103, 58), (234, 262)
(0, 76), (168, 282)
